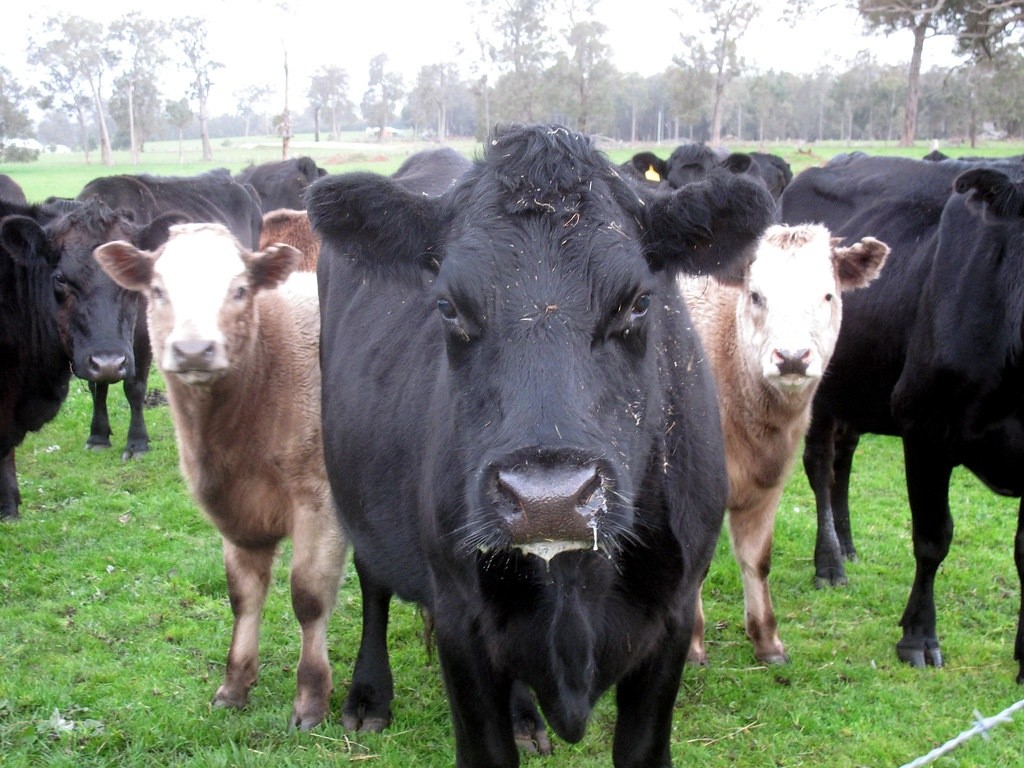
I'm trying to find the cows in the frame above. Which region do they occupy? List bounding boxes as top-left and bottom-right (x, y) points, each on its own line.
(1, 122), (1024, 768)
